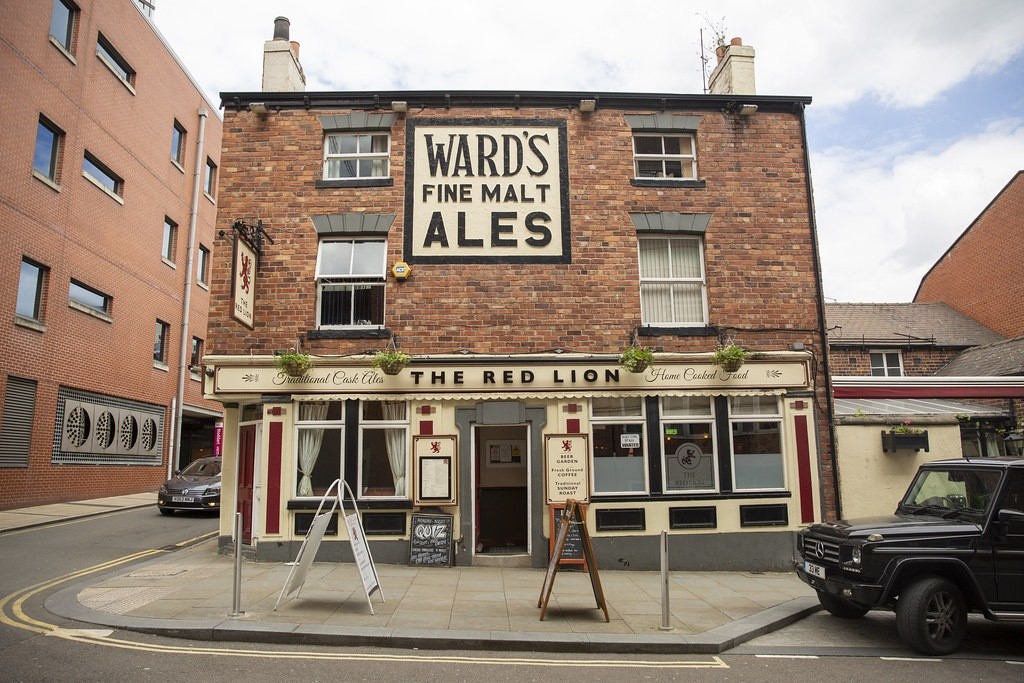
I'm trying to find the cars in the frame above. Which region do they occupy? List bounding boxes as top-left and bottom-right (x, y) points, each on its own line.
(157, 453), (224, 519)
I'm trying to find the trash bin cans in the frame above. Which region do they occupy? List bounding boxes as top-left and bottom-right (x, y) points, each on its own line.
(947, 494), (967, 507)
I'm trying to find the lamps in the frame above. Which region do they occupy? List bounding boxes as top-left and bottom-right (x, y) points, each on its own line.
(391, 100), (411, 114)
(734, 101), (760, 118)
(249, 101), (271, 114)
(579, 99), (598, 112)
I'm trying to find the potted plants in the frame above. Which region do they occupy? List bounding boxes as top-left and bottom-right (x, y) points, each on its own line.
(694, 10), (729, 65)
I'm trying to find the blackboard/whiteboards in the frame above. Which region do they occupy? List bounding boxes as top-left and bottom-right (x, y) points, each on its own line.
(550, 502), (587, 564)
(408, 506), (454, 567)
(540, 499), (606, 609)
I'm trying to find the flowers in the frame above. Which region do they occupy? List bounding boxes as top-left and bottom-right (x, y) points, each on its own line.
(715, 341), (748, 372)
(622, 343), (656, 372)
(891, 417), (916, 433)
(371, 345), (412, 369)
(274, 346), (313, 378)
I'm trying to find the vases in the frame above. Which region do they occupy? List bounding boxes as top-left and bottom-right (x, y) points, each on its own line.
(719, 358), (745, 372)
(378, 362), (406, 375)
(285, 363), (310, 377)
(881, 429), (933, 453)
(630, 360), (649, 373)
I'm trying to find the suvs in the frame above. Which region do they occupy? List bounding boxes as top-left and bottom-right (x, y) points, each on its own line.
(792, 456), (1024, 658)
